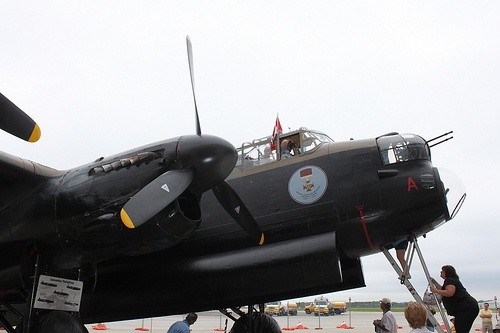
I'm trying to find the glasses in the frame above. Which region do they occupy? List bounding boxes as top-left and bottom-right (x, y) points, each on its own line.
(380, 302), (382, 304)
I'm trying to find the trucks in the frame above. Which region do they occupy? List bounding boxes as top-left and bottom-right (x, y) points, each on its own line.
(264, 301), (298, 316)
(304, 295), (347, 316)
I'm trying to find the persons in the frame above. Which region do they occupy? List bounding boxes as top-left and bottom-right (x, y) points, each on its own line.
(450, 318), (456, 333)
(373, 297), (398, 333)
(287, 141), (301, 156)
(395, 239), (411, 279)
(404, 301), (436, 333)
(281, 139), (298, 159)
(480, 303), (493, 333)
(496, 306), (500, 323)
(167, 313), (198, 333)
(430, 265), (479, 333)
(327, 302), (335, 315)
(261, 145), (275, 161)
(406, 136), (429, 160)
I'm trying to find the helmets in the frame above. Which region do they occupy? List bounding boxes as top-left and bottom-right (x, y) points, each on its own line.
(281, 139), (291, 150)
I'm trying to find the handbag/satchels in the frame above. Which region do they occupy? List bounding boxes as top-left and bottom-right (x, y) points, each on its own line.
(423, 282), (441, 305)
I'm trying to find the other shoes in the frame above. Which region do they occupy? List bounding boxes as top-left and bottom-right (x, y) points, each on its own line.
(398, 273), (411, 279)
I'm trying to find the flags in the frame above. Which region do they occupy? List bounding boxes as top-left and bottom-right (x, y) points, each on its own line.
(271, 117), (282, 150)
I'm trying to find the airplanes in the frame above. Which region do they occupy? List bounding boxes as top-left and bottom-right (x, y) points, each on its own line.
(0, 36), (466, 333)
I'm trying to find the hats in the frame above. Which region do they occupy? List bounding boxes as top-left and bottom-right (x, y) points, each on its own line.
(188, 313), (196, 321)
(497, 306), (500, 309)
(450, 318), (455, 321)
(381, 298), (390, 305)
